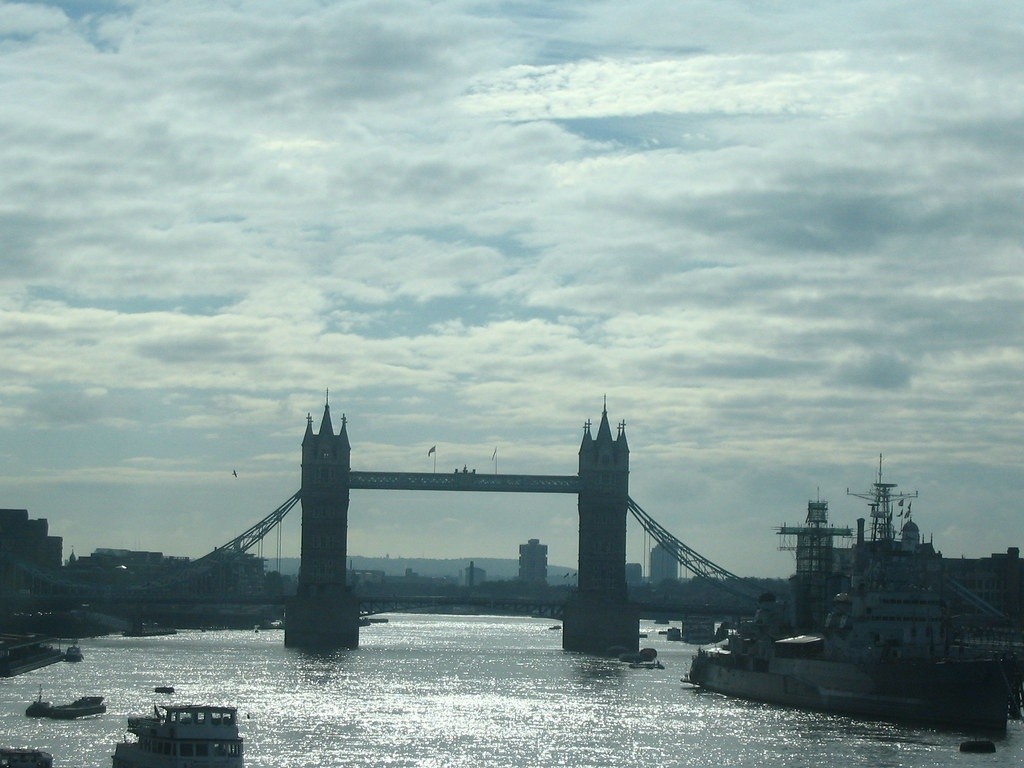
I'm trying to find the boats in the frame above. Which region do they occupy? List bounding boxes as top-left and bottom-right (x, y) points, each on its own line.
(690, 515), (1013, 731)
(64, 638), (87, 664)
(110, 700), (245, 768)
(25, 683), (107, 720)
(0, 748), (53, 768)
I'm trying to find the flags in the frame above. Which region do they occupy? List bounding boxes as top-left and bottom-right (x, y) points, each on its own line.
(563, 572), (577, 579)
(491, 447), (496, 460)
(898, 500), (911, 518)
(428, 445), (436, 456)
